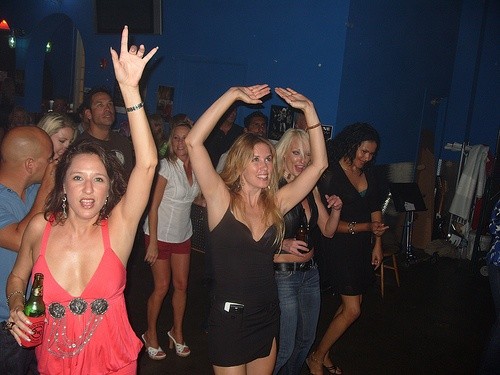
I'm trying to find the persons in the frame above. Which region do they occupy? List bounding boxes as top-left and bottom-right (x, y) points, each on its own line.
(6, 26), (158, 375)
(0, 86), (167, 375)
(272, 129), (342, 375)
(305, 123), (389, 375)
(142, 117), (206, 361)
(184, 85), (329, 375)
(147, 102), (268, 174)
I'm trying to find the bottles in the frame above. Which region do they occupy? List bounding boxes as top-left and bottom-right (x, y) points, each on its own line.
(23, 273), (46, 348)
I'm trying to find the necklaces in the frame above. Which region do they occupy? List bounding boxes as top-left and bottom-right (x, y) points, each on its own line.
(342, 166), (357, 171)
(283, 170), (296, 181)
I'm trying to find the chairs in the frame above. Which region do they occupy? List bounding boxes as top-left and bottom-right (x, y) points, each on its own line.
(371, 211), (406, 295)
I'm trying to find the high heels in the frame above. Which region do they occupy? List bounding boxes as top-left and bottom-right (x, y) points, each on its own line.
(168, 331), (191, 357)
(304, 353), (343, 375)
(141, 334), (166, 360)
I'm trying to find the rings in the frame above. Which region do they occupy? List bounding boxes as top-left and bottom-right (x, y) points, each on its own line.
(6, 321), (16, 330)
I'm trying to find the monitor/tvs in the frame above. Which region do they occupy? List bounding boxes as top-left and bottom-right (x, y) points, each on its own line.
(92, 0), (163, 35)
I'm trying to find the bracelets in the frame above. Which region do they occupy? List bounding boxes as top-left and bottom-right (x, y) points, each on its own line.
(125, 101), (145, 112)
(7, 291), (25, 307)
(348, 221), (357, 235)
(307, 123), (321, 130)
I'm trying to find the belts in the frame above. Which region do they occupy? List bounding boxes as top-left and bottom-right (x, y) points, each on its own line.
(274, 259), (315, 271)
